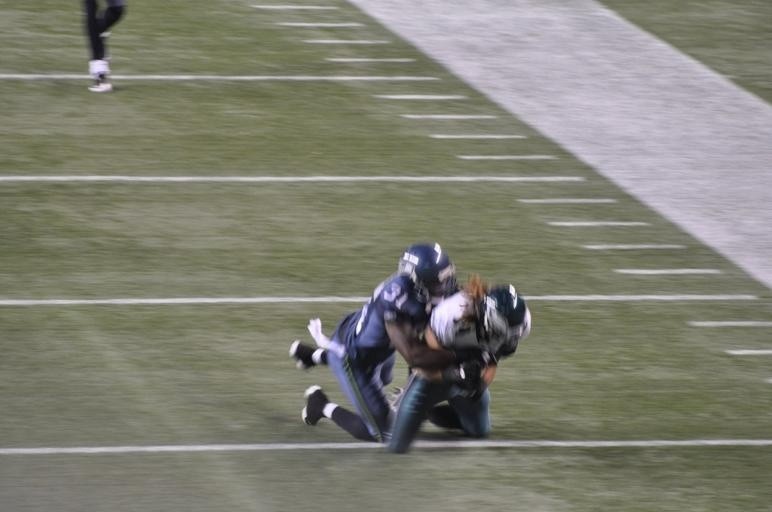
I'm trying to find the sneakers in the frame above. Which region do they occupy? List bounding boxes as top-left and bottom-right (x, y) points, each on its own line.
(301, 385), (328, 425)
(290, 340), (315, 371)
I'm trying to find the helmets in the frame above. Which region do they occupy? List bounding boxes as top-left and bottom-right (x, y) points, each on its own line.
(483, 291), (531, 341)
(399, 241), (456, 296)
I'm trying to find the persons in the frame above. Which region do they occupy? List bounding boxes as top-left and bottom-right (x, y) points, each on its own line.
(82, 0), (128, 93)
(380, 282), (533, 456)
(290, 241), (489, 446)
